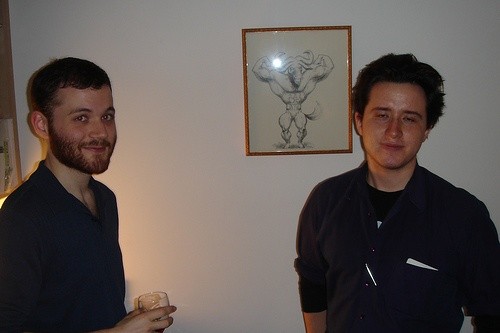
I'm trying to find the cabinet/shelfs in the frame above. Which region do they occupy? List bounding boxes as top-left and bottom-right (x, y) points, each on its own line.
(0, 0), (23, 200)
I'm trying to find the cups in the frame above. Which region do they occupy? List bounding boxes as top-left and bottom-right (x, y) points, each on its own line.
(139, 292), (170, 333)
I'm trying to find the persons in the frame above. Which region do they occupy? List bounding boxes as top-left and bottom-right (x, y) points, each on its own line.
(2, 56), (178, 333)
(293, 52), (499, 333)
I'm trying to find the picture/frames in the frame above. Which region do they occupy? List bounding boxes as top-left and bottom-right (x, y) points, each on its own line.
(241, 25), (353, 156)
